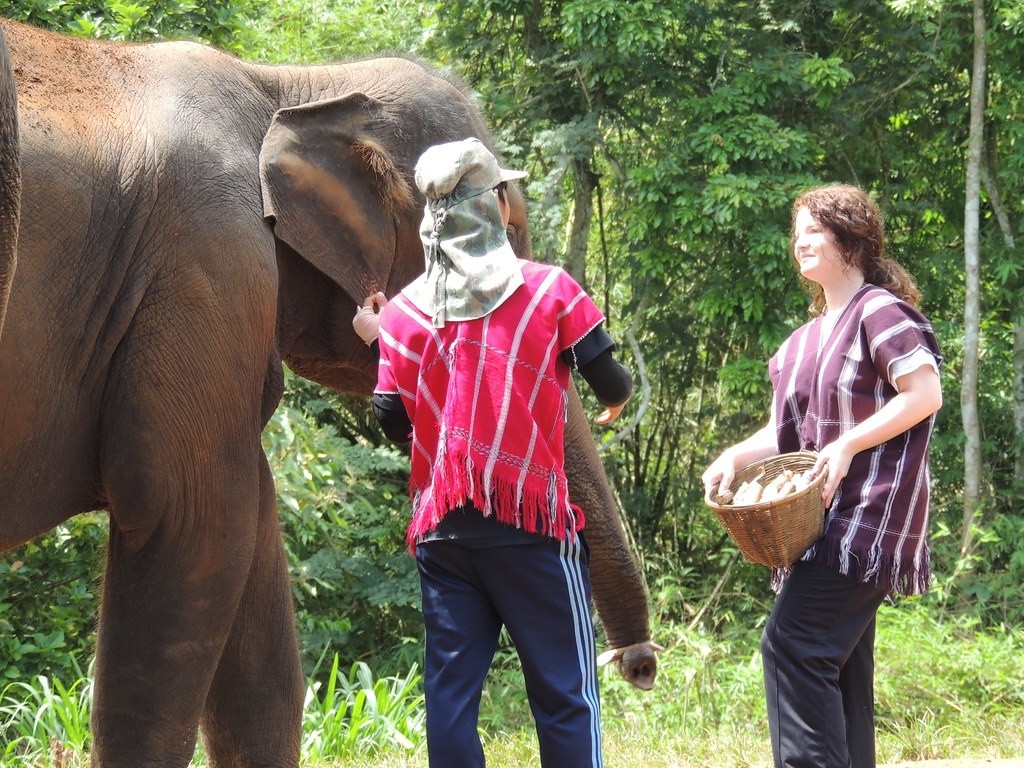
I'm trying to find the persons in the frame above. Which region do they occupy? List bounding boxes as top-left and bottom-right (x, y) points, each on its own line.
(351, 136), (632, 768)
(702, 186), (944, 768)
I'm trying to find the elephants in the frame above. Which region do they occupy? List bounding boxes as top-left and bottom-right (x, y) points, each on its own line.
(0, 19), (659, 768)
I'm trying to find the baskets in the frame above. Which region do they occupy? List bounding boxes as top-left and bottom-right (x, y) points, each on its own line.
(705, 451), (828, 566)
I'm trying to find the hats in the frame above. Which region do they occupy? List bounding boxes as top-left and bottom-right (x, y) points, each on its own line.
(401, 137), (528, 328)
